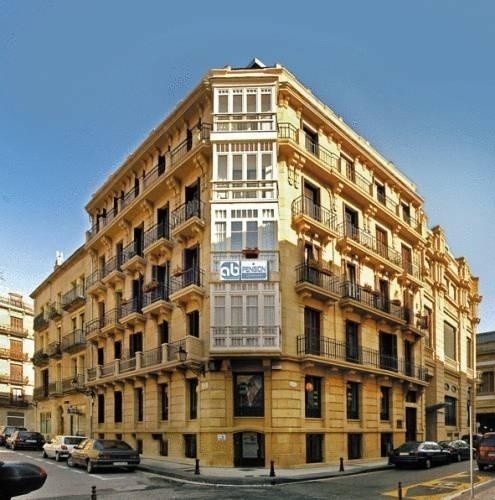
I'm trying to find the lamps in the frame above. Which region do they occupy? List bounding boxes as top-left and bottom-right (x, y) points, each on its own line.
(174, 346), (205, 378)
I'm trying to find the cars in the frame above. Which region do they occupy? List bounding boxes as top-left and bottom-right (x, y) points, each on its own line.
(67, 438), (140, 473)
(0, 426), (45, 451)
(388, 431), (495, 471)
(42, 435), (89, 462)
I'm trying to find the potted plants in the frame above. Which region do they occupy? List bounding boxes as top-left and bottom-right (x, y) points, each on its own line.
(120, 298), (128, 305)
(172, 265), (184, 277)
(142, 280), (159, 293)
(362, 284), (372, 293)
(242, 246), (260, 254)
